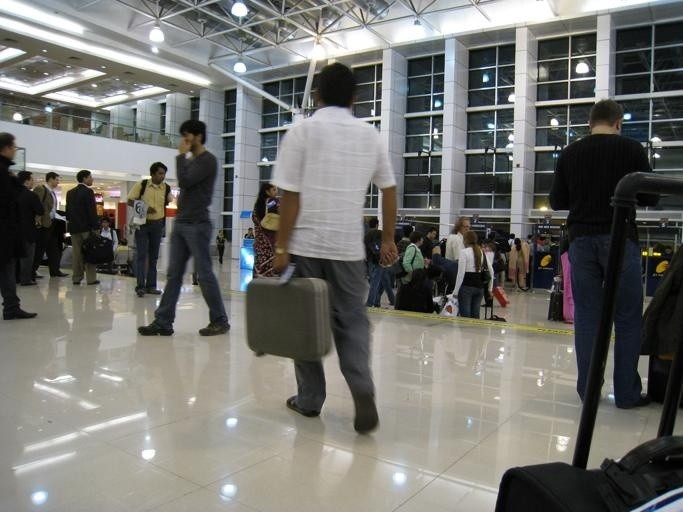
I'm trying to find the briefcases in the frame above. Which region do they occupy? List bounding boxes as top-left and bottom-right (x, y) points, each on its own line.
(245, 265), (332, 362)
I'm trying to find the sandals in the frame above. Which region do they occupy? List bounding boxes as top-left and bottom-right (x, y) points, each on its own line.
(286, 394), (320, 417)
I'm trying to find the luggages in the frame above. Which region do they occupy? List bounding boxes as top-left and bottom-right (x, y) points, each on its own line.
(493, 286), (511, 307)
(484, 303), (506, 321)
(547, 273), (564, 320)
(493, 169), (683, 511)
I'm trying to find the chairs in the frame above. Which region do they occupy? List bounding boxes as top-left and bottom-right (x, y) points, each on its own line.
(76, 124), (170, 148)
(111, 251), (132, 275)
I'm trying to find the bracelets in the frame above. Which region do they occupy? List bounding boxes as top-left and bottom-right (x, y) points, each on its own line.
(274, 247), (287, 254)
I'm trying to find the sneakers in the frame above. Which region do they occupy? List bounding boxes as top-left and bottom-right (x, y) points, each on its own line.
(3, 270), (230, 336)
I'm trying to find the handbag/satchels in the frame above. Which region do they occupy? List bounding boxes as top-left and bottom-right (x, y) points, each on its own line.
(481, 265), (491, 288)
(390, 254), (409, 279)
(438, 293), (459, 317)
(260, 213), (280, 231)
(81, 232), (115, 265)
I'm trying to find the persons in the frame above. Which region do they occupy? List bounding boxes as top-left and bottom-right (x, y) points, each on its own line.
(132, 119), (234, 339)
(364, 216), (547, 319)
(65, 169), (103, 285)
(8, 170), (16, 176)
(255, 61), (398, 436)
(552, 96), (658, 410)
(192, 262), (200, 285)
(14, 170), (43, 285)
(252, 181), (282, 279)
(216, 230), (227, 265)
(0, 132), (37, 321)
(126, 162), (172, 295)
(28, 172), (68, 279)
(244, 227), (254, 239)
(98, 216), (119, 274)
(114, 239), (128, 257)
